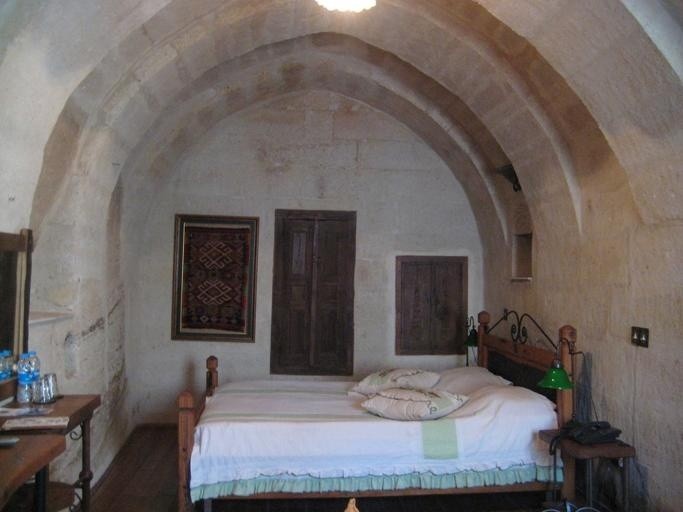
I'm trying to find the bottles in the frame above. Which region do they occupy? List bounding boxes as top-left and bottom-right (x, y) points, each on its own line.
(17, 354), (32, 403)
(29, 351), (40, 396)
(0, 351), (14, 379)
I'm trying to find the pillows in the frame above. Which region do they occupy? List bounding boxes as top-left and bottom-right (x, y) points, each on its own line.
(349, 365), (471, 423)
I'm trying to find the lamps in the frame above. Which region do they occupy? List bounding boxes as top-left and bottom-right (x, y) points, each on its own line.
(464, 315), (479, 366)
(536, 336), (601, 427)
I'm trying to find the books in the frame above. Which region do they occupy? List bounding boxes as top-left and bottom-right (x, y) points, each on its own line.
(2, 417), (68, 431)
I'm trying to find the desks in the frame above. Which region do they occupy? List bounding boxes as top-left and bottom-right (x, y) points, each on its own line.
(539, 429), (635, 512)
(0, 393), (103, 512)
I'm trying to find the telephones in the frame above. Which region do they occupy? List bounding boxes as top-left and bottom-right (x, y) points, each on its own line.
(569, 421), (622, 445)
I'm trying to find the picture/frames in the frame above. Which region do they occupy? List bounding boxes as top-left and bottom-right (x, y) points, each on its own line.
(171, 212), (259, 343)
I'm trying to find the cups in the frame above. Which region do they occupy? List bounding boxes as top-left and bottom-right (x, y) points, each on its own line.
(45, 373), (60, 397)
(34, 377), (50, 401)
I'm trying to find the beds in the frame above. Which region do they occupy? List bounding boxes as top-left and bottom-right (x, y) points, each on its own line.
(175, 308), (578, 511)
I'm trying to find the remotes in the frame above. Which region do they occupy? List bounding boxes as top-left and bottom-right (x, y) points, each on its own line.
(0, 437), (20, 447)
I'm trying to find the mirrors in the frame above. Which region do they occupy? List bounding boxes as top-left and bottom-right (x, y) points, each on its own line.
(1, 225), (34, 389)
